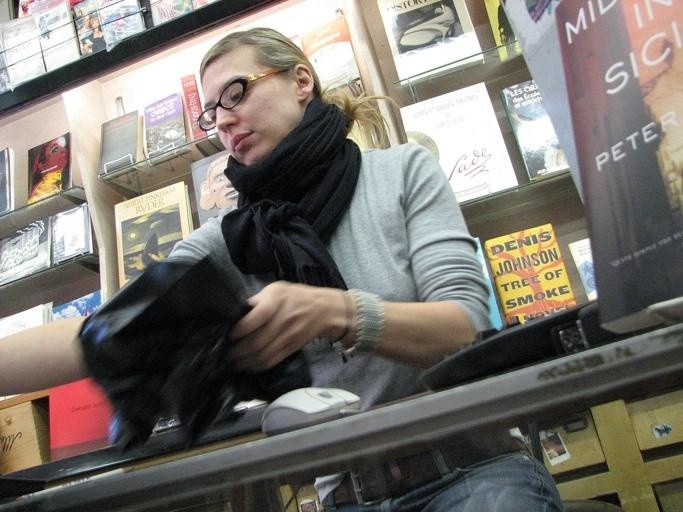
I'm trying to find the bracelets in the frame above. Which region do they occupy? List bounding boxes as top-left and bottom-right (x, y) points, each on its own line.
(334, 291), (351, 344)
(344, 286), (385, 362)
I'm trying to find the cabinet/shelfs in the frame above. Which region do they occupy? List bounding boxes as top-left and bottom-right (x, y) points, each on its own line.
(0, 1), (598, 479)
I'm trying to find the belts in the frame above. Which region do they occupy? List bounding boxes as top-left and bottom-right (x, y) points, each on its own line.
(312, 432), (534, 505)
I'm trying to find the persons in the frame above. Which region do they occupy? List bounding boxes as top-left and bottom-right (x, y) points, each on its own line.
(1, 26), (565, 512)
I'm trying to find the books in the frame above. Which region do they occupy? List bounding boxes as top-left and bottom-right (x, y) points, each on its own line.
(480, 223), (577, 325)
(191, 149), (238, 227)
(492, 75), (572, 182)
(294, 10), (372, 98)
(377, 2), (489, 80)
(2, 290), (102, 338)
(472, 236), (504, 331)
(394, 80), (520, 213)
(2, 201), (99, 284)
(2, 401), (46, 474)
(485, 1), (530, 65)
(47, 378), (115, 462)
(28, 132), (72, 208)
(1, 148), (15, 212)
(0, 0), (212, 92)
(114, 180), (193, 289)
(98, 74), (226, 192)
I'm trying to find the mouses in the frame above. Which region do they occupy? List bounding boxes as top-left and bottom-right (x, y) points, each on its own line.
(261, 386), (361, 434)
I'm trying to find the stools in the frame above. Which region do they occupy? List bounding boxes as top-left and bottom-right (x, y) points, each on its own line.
(560, 500), (627, 512)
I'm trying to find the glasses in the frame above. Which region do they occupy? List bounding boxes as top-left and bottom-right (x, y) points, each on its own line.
(195, 64), (292, 132)
